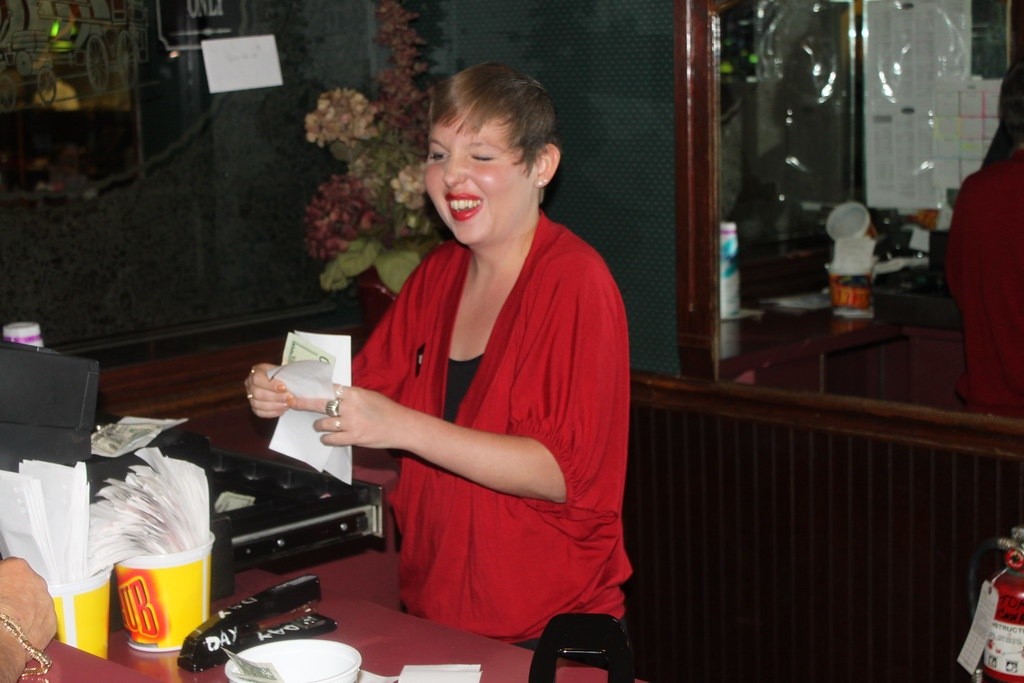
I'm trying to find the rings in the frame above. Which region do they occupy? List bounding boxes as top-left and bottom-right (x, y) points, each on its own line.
(246, 394), (253, 399)
(251, 369), (256, 374)
(336, 417), (342, 432)
(336, 386), (343, 399)
(326, 400), (341, 417)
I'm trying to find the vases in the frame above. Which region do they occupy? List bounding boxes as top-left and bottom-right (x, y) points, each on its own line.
(354, 272), (399, 331)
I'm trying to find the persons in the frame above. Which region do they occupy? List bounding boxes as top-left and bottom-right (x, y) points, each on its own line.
(0, 556), (58, 682)
(244, 57), (634, 669)
(946, 55), (1024, 420)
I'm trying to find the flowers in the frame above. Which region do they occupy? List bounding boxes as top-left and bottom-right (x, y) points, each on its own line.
(301, 0), (440, 260)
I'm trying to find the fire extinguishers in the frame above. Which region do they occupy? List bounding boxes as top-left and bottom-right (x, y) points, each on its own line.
(981, 527), (1024, 683)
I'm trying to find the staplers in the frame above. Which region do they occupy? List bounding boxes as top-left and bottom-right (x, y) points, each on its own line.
(176, 574), (337, 673)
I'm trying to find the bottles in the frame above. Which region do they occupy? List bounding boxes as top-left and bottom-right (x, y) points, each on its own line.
(720, 222), (745, 322)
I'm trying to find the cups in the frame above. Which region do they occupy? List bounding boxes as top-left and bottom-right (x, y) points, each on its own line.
(825, 262), (878, 321)
(826, 204), (879, 247)
(43, 575), (110, 660)
(117, 529), (220, 652)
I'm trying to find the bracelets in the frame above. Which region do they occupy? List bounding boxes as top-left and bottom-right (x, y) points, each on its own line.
(0, 613), (52, 675)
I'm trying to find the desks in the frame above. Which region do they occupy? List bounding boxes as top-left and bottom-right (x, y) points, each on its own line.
(0, 567), (649, 683)
(717, 284), (1024, 421)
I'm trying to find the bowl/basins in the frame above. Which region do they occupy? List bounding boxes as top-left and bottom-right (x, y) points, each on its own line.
(226, 639), (362, 683)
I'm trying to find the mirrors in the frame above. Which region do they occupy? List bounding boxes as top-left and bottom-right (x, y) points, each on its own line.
(675, 0), (1024, 460)
